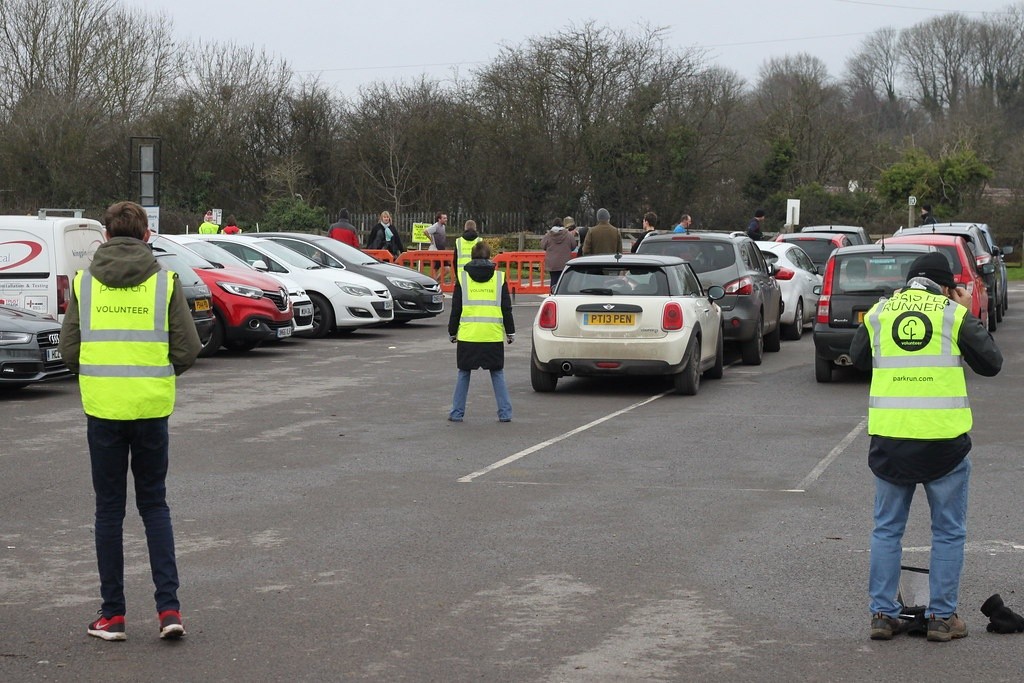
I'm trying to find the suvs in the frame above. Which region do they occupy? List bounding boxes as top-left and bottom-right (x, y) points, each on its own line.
(634, 228), (784, 366)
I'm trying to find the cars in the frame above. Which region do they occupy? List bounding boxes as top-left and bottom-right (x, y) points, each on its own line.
(141, 232), (445, 358)
(0, 305), (77, 385)
(530, 251), (725, 396)
(753, 222), (1014, 382)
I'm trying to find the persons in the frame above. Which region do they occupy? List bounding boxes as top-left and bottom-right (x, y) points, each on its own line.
(747, 209), (765, 240)
(327, 208), (360, 249)
(581, 208), (622, 255)
(921, 204), (938, 224)
(850, 252), (1004, 641)
(366, 211), (404, 262)
(59, 201), (203, 641)
(630, 212), (658, 253)
(198, 210), (241, 235)
(447, 242), (516, 422)
(452, 219), (484, 278)
(541, 217), (576, 288)
(674, 214), (692, 233)
(424, 213), (449, 270)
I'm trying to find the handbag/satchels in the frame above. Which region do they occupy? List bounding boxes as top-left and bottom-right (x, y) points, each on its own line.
(428, 240), (438, 251)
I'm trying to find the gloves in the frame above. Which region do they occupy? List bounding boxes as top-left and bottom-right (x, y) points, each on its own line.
(980, 593), (1024, 633)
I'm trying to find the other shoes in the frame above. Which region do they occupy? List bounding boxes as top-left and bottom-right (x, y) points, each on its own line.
(500, 419), (511, 422)
(448, 416), (453, 420)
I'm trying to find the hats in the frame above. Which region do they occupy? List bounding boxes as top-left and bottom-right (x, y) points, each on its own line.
(339, 208), (349, 219)
(203, 211), (213, 222)
(906, 252), (957, 288)
(596, 208), (611, 222)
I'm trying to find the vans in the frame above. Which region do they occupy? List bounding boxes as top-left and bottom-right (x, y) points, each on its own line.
(0, 208), (109, 326)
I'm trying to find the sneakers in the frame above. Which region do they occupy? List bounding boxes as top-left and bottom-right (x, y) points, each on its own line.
(159, 611), (186, 638)
(88, 609), (126, 639)
(869, 611), (901, 638)
(927, 612), (969, 641)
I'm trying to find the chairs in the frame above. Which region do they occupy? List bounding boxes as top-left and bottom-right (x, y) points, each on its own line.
(845, 260), (868, 291)
(634, 274), (658, 294)
(582, 272), (606, 289)
(901, 261), (912, 279)
